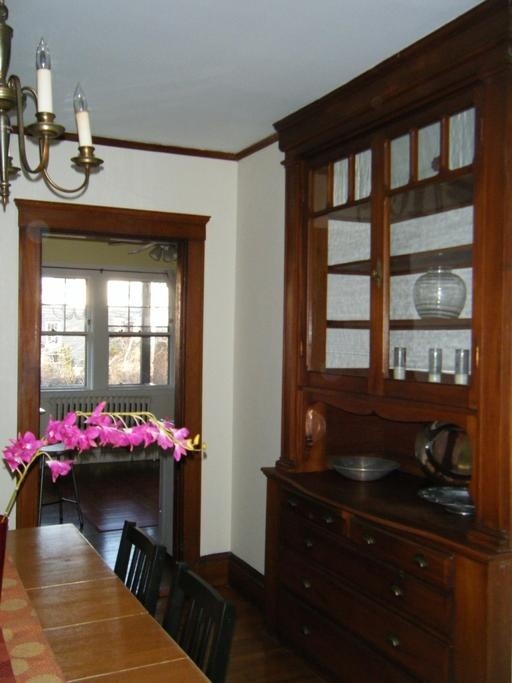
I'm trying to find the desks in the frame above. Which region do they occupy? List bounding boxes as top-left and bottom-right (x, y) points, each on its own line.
(0, 522), (217, 683)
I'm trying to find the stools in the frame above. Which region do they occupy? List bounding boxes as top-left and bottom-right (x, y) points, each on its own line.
(38, 443), (85, 529)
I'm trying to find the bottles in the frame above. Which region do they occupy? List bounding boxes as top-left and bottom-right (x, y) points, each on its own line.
(412, 252), (467, 319)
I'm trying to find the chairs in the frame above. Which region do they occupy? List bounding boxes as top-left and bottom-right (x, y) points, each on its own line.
(112, 519), (237, 682)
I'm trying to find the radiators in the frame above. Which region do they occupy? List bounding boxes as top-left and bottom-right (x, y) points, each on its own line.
(56, 398), (152, 429)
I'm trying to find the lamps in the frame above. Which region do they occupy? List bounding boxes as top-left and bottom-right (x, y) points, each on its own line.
(1, 3), (102, 219)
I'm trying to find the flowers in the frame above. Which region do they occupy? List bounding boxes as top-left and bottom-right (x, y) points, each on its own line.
(2, 405), (208, 516)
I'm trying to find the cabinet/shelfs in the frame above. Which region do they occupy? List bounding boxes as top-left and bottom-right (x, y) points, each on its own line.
(263, 477), (458, 682)
(291, 57), (489, 411)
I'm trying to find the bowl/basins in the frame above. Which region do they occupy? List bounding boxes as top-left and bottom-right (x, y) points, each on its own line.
(328, 456), (401, 482)
